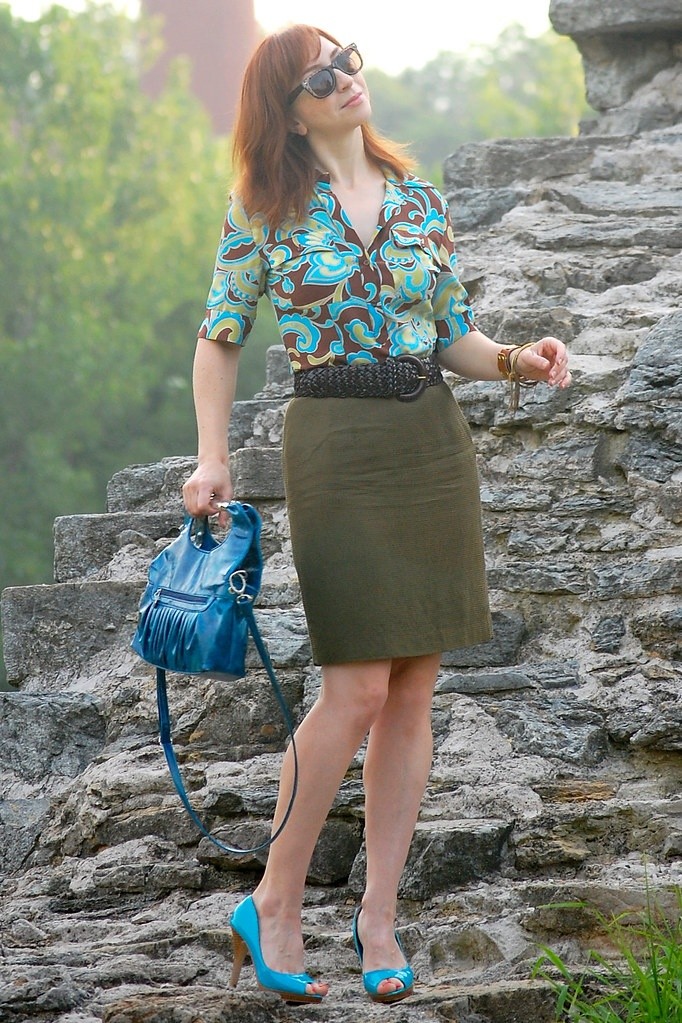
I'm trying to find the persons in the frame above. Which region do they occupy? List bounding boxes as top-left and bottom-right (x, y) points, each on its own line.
(180, 21), (571, 1005)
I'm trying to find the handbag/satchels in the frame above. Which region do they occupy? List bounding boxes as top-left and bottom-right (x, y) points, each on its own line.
(131, 496), (265, 679)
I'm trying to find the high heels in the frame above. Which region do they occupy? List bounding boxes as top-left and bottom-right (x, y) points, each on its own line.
(229, 895), (326, 1004)
(351, 905), (415, 1002)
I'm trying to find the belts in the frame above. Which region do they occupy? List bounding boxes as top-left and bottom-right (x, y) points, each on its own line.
(293, 355), (446, 402)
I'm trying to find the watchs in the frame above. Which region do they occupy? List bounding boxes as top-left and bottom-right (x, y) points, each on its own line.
(497, 339), (535, 382)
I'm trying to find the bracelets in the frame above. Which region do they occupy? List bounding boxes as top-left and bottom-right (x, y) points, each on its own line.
(508, 341), (543, 414)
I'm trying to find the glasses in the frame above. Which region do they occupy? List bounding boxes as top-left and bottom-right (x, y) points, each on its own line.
(285, 43), (363, 107)
